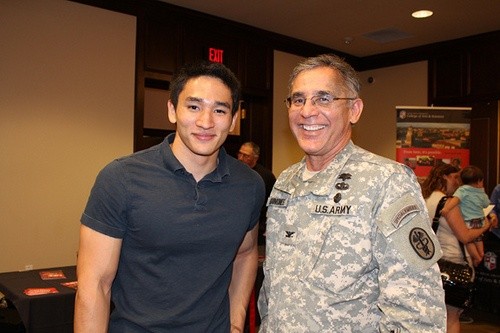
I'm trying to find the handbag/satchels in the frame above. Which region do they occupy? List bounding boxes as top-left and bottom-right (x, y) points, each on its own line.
(437, 258), (472, 309)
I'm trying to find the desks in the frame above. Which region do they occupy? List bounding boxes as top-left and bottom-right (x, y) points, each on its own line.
(0, 264), (78, 333)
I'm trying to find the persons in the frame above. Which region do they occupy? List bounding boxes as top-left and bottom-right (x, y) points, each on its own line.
(483, 182), (500, 292)
(413, 159), (492, 332)
(439, 163), (492, 268)
(75, 63), (266, 333)
(257, 53), (447, 333)
(238, 140), (278, 248)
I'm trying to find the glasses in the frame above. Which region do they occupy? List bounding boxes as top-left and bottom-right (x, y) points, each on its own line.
(237, 152), (250, 158)
(284, 94), (357, 110)
